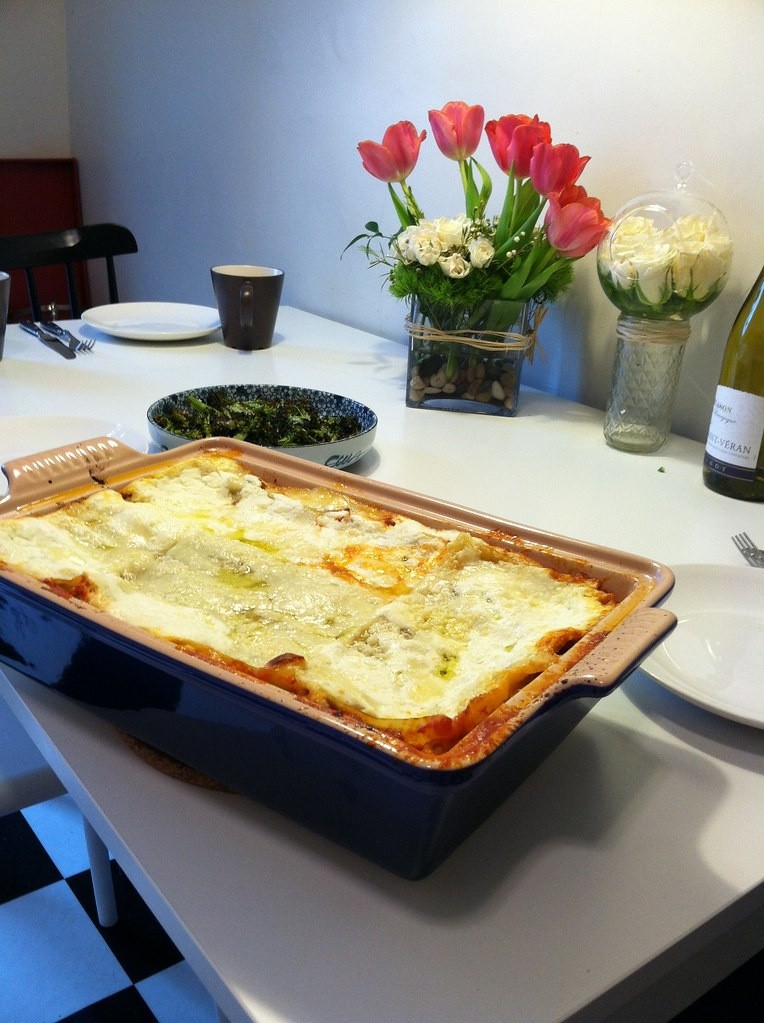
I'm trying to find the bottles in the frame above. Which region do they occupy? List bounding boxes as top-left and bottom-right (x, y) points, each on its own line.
(703, 264), (764, 503)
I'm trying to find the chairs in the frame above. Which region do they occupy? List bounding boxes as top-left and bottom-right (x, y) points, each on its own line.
(0, 224), (138, 322)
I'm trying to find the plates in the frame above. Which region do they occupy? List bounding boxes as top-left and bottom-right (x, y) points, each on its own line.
(81, 302), (222, 340)
(636, 563), (764, 730)
(147, 384), (378, 470)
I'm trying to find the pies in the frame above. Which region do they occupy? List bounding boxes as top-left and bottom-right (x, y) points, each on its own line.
(0, 453), (618, 750)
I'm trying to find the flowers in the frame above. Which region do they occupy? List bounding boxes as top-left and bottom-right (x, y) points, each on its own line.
(597, 213), (734, 318)
(340, 101), (613, 378)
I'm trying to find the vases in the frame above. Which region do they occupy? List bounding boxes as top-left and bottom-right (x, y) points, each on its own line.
(596, 162), (735, 454)
(406, 293), (530, 417)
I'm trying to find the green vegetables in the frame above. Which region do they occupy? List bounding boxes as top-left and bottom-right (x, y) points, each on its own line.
(154, 388), (363, 449)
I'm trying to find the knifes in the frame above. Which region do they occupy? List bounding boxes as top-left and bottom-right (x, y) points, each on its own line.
(19, 319), (77, 359)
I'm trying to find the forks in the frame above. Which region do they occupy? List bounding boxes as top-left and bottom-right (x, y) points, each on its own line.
(40, 319), (96, 351)
(730, 532), (764, 569)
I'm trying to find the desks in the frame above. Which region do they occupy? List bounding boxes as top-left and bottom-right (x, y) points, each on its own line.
(0, 306), (764, 1023)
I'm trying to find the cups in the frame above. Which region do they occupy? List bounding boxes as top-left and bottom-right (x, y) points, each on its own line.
(211, 265), (285, 350)
(0, 271), (11, 361)
